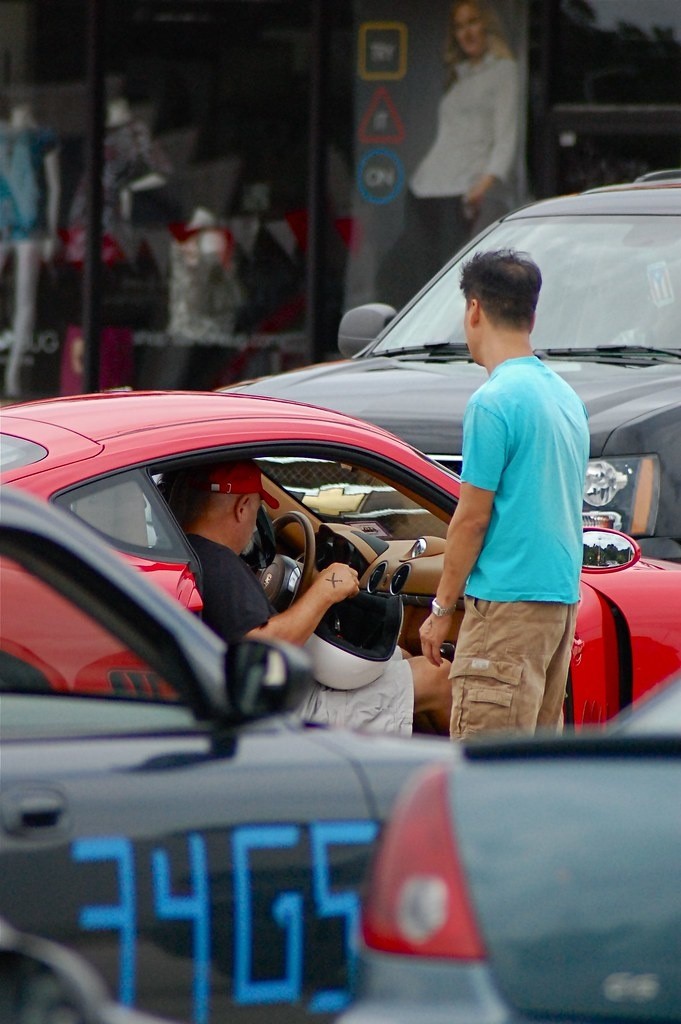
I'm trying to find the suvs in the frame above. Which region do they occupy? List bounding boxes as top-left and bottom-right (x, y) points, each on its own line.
(213, 166), (681, 572)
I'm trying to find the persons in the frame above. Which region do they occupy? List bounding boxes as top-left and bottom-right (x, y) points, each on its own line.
(0, 101), (249, 397)
(419, 248), (591, 744)
(375, 0), (521, 308)
(156, 458), (452, 735)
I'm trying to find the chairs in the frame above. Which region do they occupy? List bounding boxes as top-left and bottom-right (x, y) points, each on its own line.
(72, 469), (147, 549)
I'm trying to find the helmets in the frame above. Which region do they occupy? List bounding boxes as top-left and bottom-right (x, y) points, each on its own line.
(304, 592), (404, 690)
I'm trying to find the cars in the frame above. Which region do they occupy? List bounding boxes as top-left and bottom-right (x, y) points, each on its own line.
(0, 489), (462, 1024)
(0, 387), (681, 742)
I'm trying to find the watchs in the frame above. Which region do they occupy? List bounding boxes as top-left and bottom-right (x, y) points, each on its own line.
(432, 598), (457, 617)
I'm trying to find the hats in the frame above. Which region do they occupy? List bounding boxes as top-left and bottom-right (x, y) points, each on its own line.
(177, 459), (279, 509)
